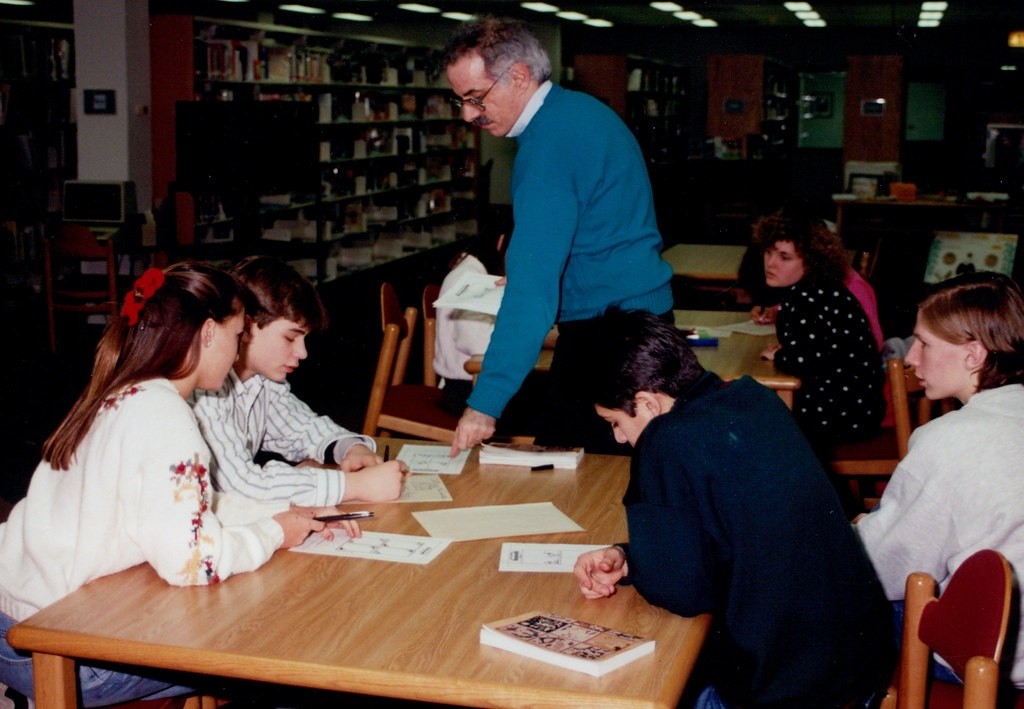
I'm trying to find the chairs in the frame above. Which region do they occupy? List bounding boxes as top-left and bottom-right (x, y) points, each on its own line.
(877, 549), (1014, 709)
(362, 280), (442, 437)
(827, 359), (955, 524)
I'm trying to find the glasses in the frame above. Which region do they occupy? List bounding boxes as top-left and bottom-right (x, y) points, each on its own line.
(449, 61), (530, 112)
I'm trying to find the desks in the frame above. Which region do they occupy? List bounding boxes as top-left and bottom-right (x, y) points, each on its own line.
(6, 434), (712, 709)
(462, 309), (801, 412)
(830, 194), (1006, 237)
(661, 243), (857, 304)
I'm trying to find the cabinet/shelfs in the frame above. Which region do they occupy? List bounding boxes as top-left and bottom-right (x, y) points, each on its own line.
(1, 22), (482, 319)
(43, 211), (145, 352)
(574, 53), (904, 246)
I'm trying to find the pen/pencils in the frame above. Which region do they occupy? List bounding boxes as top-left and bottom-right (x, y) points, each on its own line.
(313, 512), (374, 522)
(384, 445), (389, 462)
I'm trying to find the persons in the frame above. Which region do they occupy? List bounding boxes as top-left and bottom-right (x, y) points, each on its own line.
(0, 262), (363, 709)
(848, 269), (1024, 689)
(740, 197), (888, 459)
(573, 308), (901, 709)
(184, 254), (412, 508)
(438, 14), (676, 459)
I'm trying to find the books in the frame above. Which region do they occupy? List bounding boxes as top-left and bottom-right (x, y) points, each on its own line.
(205, 40), (326, 83)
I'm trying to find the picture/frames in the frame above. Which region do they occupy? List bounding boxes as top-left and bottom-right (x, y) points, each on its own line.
(810, 92), (833, 118)
